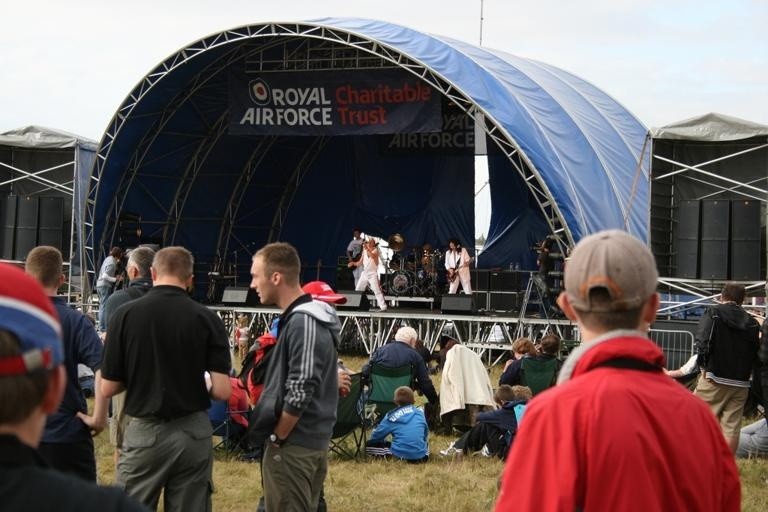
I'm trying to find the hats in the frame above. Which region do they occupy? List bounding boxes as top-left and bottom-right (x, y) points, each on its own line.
(0, 261), (65, 376)
(563, 229), (661, 313)
(300, 281), (346, 306)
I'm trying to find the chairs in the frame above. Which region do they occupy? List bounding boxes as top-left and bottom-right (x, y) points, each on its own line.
(327, 372), (368, 461)
(364, 360), (415, 427)
(520, 358), (557, 396)
(206, 395), (230, 460)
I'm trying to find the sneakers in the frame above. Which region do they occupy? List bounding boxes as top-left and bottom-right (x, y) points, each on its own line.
(439, 442), (465, 458)
(480, 443), (494, 458)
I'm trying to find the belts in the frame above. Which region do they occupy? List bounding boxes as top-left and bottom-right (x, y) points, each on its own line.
(129, 415), (188, 425)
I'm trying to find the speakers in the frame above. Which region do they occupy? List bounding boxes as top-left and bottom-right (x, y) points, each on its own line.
(335, 290), (371, 312)
(677, 198), (762, 290)
(0, 193), (65, 262)
(441, 294), (478, 315)
(222, 287), (257, 306)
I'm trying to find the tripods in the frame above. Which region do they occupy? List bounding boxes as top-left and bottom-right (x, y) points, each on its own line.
(380, 249), (441, 295)
(519, 276), (549, 319)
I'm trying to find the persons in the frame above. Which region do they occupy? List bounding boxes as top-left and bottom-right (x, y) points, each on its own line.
(0, 247), (142, 512)
(347, 234), (472, 315)
(493, 231), (741, 512)
(361, 321), (562, 465)
(659, 280), (767, 458)
(235, 243), (355, 512)
(95, 243), (232, 512)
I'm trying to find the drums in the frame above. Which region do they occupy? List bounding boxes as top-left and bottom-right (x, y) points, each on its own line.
(390, 270), (415, 294)
